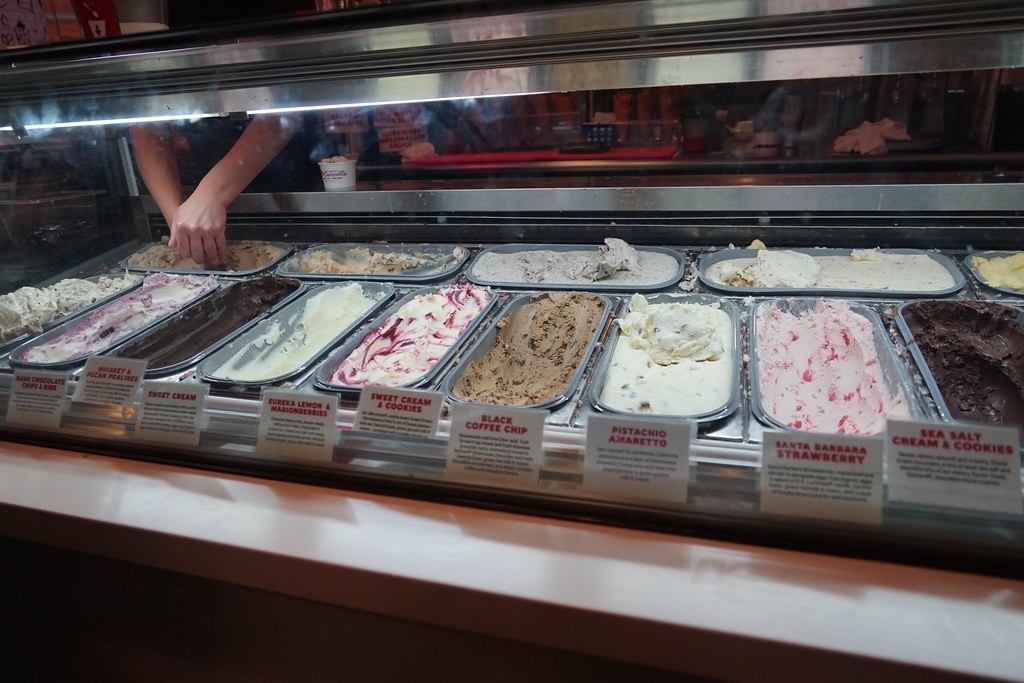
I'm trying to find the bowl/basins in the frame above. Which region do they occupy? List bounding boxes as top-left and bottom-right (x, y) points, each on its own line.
(318, 160), (357, 189)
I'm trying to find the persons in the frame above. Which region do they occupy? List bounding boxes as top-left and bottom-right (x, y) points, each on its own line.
(128, 0), (325, 265)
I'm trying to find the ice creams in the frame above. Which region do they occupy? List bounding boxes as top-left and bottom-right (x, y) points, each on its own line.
(318, 155), (353, 163)
